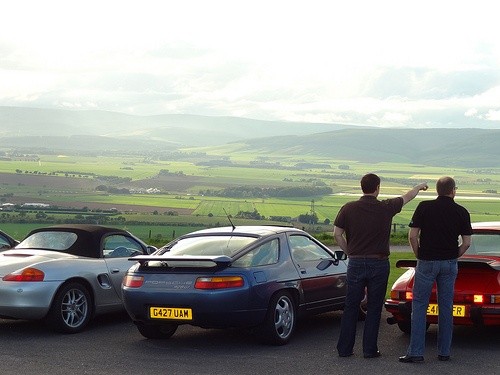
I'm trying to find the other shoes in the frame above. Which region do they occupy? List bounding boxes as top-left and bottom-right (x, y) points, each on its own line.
(437, 354), (449, 361)
(398, 354), (425, 363)
(364, 351), (381, 357)
(339, 352), (354, 357)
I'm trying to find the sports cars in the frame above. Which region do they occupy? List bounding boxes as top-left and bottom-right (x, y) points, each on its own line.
(0, 222), (162, 333)
(384, 222), (500, 336)
(119, 225), (370, 345)
(0, 229), (20, 254)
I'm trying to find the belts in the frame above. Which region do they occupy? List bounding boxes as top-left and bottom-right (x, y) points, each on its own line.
(349, 254), (388, 259)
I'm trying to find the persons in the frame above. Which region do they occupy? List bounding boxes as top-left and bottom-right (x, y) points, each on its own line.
(334, 173), (429, 358)
(398, 176), (474, 363)
